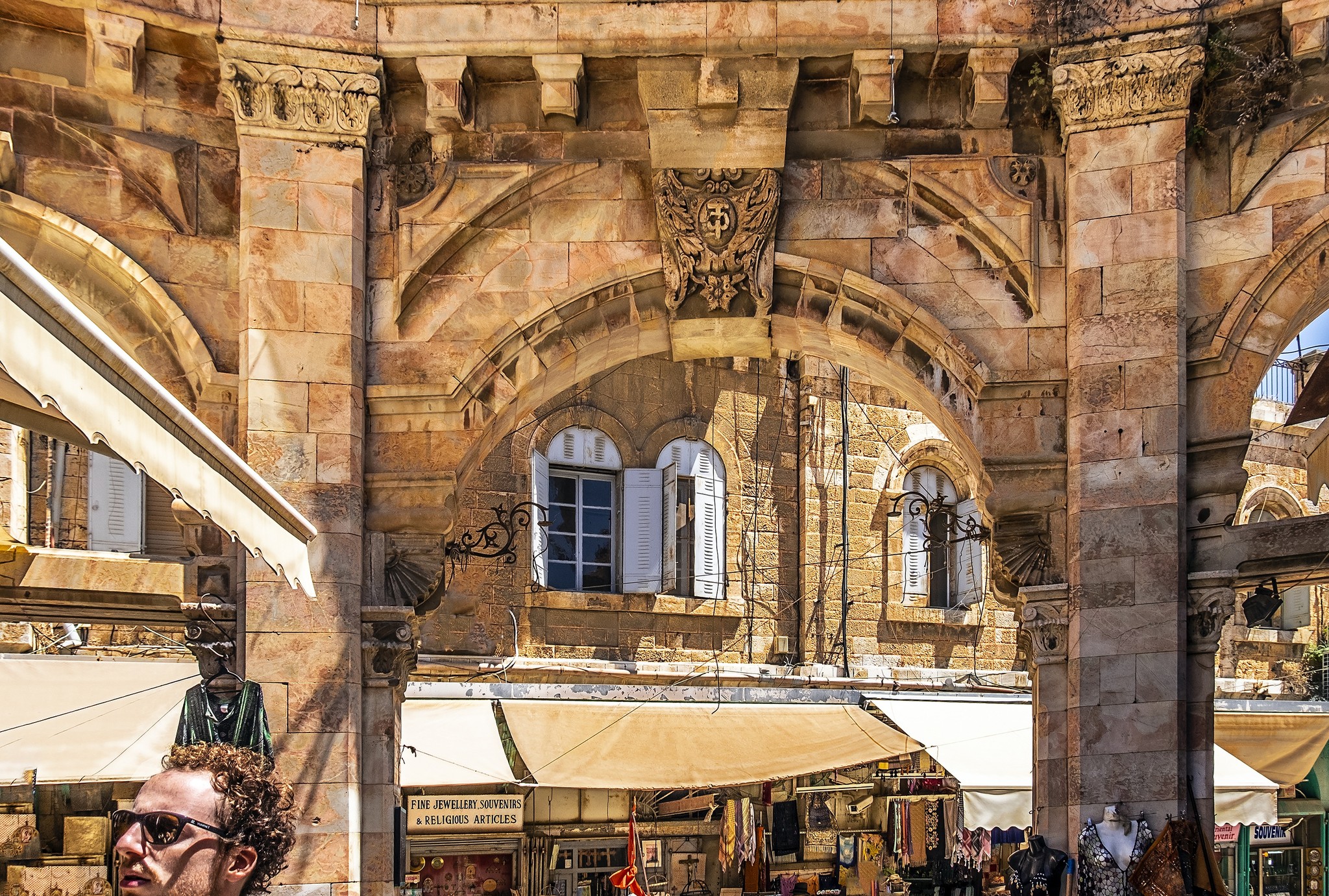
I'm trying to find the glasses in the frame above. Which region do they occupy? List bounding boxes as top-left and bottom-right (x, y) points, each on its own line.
(111, 809), (230, 845)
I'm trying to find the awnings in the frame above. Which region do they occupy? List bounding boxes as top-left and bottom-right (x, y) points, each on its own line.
(498, 700), (935, 788)
(871, 699), (1280, 831)
(398, 699), (520, 789)
(0, 652), (198, 788)
(0, 236), (318, 604)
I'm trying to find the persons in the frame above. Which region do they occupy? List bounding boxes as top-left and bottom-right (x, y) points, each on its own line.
(683, 861), (696, 882)
(1077, 805), (1155, 896)
(112, 742), (299, 896)
(1007, 834), (1068, 896)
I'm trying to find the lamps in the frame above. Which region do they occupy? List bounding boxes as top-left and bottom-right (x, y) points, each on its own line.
(872, 773), (954, 779)
(1242, 576), (1285, 628)
(794, 783), (874, 793)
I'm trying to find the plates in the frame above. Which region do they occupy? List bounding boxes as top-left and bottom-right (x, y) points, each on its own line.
(431, 857), (444, 869)
(410, 856), (425, 872)
(483, 879), (497, 892)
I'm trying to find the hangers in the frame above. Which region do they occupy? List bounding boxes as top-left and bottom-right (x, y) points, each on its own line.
(186, 653), (260, 699)
